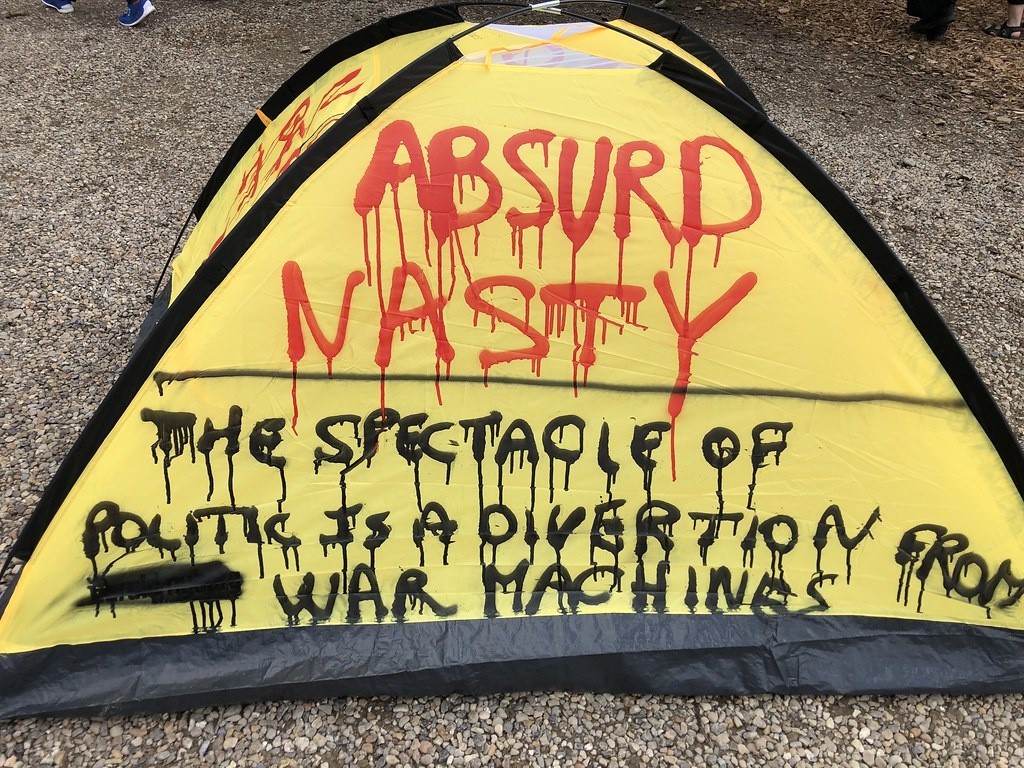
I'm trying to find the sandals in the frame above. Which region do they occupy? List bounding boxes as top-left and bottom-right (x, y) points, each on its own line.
(982, 20), (1024, 40)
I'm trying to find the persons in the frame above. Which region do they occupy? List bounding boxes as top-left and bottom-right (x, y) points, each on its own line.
(982, 0), (1024, 39)
(42, 0), (157, 26)
(905, 0), (957, 40)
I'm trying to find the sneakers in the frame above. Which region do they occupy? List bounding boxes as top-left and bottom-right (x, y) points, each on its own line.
(41, 0), (74, 14)
(118, 0), (156, 26)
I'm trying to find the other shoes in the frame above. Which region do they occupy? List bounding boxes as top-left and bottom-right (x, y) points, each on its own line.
(911, 13), (954, 42)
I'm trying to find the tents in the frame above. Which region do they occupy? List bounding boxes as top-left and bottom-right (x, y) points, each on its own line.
(0, 0), (1024, 720)
